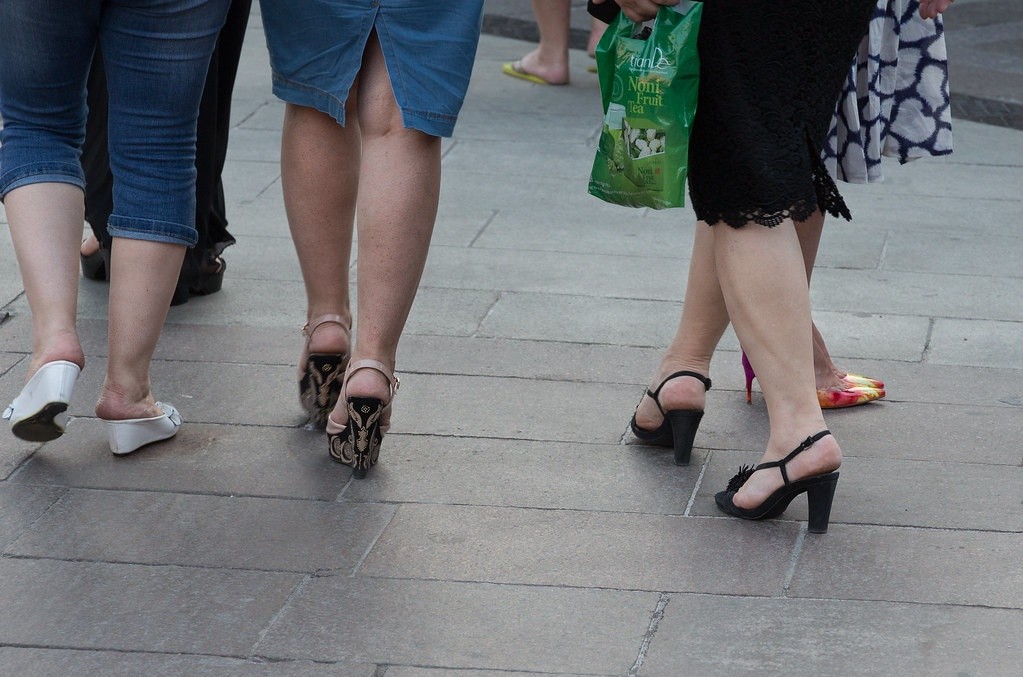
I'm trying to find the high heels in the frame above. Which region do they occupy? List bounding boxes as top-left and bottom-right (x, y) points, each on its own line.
(740, 344), (886, 409)
(839, 371), (885, 389)
(79, 237), (111, 281)
(101, 401), (182, 456)
(2, 360), (81, 441)
(170, 257), (227, 307)
(632, 371), (712, 466)
(325, 356), (401, 479)
(715, 431), (843, 533)
(298, 314), (352, 431)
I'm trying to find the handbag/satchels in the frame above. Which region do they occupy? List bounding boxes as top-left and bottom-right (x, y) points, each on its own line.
(588, 0), (703, 212)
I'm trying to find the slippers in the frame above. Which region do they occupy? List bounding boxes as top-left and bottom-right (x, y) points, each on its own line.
(588, 66), (597, 73)
(502, 59), (548, 85)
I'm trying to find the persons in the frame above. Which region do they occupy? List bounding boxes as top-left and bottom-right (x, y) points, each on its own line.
(0, 0), (229, 454)
(502, 0), (621, 85)
(615, 0), (876, 535)
(78, 0), (252, 304)
(738, 0), (954, 405)
(258, 0), (486, 478)
